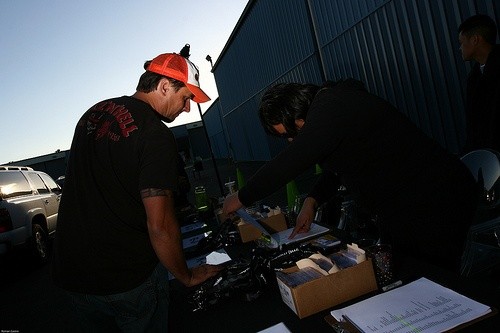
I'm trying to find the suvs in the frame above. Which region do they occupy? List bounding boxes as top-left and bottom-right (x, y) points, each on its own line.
(0, 166), (65, 259)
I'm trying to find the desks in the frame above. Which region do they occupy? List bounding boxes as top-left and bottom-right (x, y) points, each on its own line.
(167, 197), (500, 333)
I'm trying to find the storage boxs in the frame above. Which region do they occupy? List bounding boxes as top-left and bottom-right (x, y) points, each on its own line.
(236, 210), (288, 243)
(276, 249), (378, 320)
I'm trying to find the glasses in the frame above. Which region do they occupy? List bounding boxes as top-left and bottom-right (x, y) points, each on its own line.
(280, 132), (296, 140)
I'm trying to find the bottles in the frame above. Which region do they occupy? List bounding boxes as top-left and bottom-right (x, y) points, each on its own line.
(195, 186), (207, 209)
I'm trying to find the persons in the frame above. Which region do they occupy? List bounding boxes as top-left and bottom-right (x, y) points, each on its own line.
(456, 14), (500, 154)
(222, 78), (478, 285)
(53, 52), (225, 333)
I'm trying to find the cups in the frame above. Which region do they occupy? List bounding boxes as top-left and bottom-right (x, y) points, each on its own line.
(224, 180), (236, 195)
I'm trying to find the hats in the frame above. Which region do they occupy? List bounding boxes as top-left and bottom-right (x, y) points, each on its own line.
(144, 53), (211, 103)
